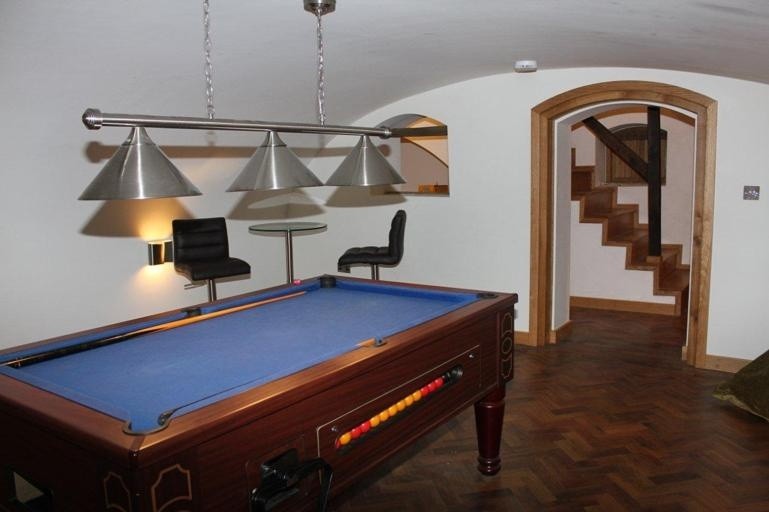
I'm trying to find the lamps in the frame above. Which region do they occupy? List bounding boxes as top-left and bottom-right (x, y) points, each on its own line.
(77, 0), (407, 200)
(147, 241), (175, 266)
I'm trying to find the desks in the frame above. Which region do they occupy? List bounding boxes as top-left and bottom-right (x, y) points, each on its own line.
(0, 275), (518, 512)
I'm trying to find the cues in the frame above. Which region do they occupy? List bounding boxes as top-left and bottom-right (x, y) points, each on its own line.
(0, 289), (309, 369)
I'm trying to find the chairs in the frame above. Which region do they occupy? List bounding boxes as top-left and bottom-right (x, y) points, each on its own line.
(251, 222), (327, 282)
(172, 217), (253, 302)
(336, 209), (407, 280)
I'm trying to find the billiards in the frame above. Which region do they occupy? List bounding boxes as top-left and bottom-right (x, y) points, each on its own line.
(333, 369), (460, 449)
